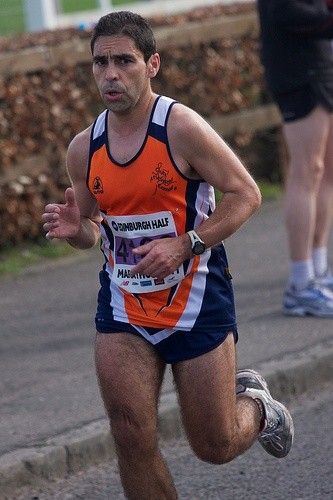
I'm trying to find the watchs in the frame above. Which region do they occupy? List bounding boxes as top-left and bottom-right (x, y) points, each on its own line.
(187, 230), (206, 256)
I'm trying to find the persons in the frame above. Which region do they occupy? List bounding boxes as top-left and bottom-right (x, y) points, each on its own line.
(255, 0), (333, 320)
(39, 10), (296, 500)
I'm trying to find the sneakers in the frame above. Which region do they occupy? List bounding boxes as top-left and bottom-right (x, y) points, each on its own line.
(281, 284), (333, 318)
(236, 369), (293, 458)
(319, 272), (333, 291)
